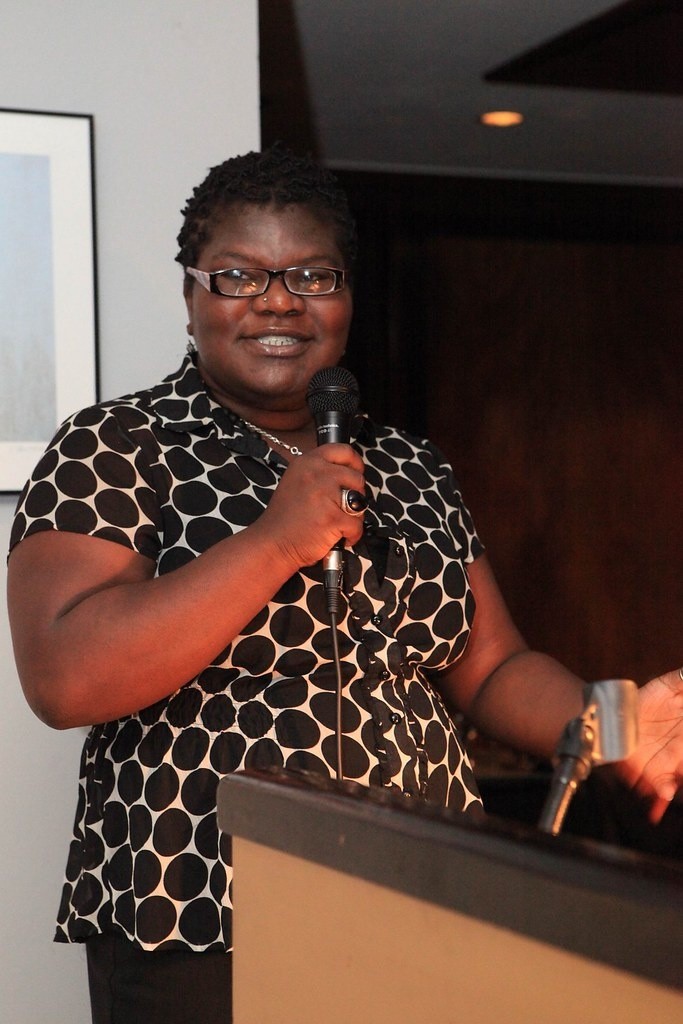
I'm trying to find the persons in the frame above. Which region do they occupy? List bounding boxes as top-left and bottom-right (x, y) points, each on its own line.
(6, 150), (683, 1024)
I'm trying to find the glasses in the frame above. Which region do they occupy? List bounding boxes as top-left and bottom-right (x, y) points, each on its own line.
(185, 265), (351, 297)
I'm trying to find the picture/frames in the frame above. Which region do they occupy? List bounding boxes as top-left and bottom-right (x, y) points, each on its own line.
(0, 106), (108, 500)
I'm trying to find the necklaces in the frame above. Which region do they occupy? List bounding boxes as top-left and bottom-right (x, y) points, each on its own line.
(240, 417), (303, 456)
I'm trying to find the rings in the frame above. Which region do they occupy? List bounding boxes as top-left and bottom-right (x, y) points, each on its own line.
(341, 489), (369, 517)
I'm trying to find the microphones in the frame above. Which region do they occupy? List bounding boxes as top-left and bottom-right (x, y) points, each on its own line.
(304, 366), (360, 610)
